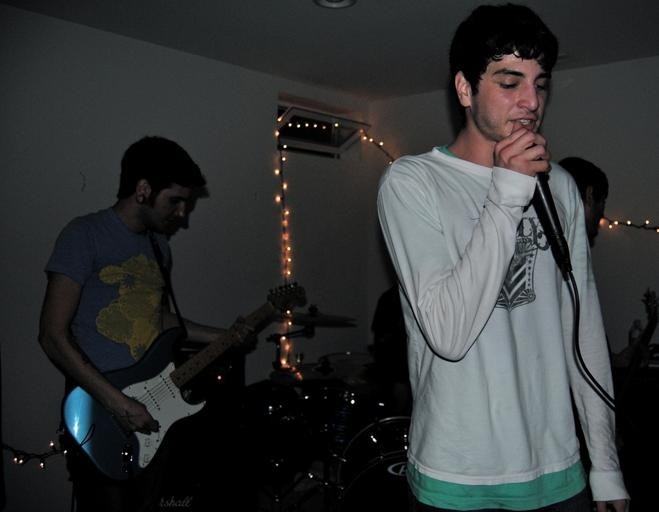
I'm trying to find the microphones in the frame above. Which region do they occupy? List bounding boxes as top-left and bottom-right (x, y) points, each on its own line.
(524, 142), (574, 273)
(266, 326), (315, 342)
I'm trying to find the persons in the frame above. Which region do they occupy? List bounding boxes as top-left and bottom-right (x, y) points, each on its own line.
(375, 1), (637, 510)
(559, 156), (651, 382)
(38, 133), (267, 512)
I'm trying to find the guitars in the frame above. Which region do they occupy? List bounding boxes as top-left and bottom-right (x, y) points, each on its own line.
(62, 281), (306, 481)
(627, 286), (659, 370)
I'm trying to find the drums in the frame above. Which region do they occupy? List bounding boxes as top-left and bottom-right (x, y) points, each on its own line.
(256, 384), (413, 512)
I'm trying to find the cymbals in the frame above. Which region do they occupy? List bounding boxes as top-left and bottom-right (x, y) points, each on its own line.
(271, 352), (372, 386)
(273, 313), (358, 328)
